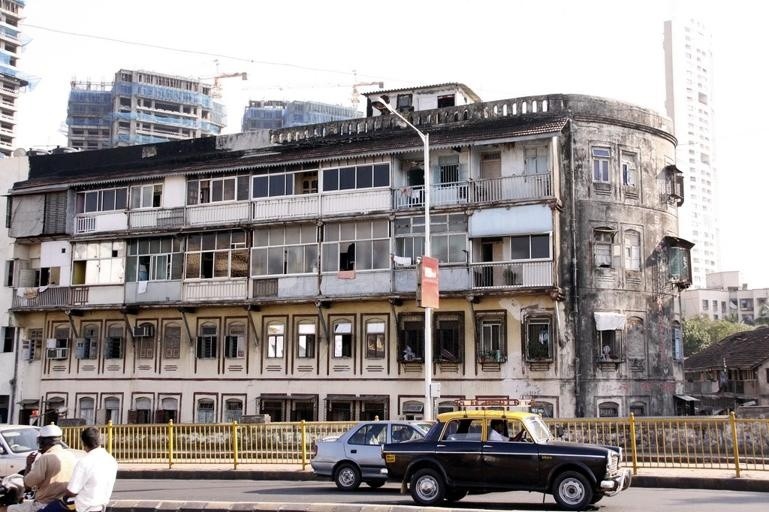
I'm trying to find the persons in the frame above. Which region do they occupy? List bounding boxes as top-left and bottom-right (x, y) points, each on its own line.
(0, 423), (76, 512)
(38, 426), (119, 512)
(484, 420), (525, 442)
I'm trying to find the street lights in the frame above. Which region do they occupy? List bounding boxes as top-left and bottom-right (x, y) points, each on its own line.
(371, 94), (435, 421)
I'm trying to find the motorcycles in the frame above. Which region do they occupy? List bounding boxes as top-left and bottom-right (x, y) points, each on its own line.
(0, 471), (77, 512)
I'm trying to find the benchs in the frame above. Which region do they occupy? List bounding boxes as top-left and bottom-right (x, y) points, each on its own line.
(349, 432), (379, 445)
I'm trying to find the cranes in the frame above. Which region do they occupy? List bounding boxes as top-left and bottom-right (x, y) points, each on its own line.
(337, 71), (384, 107)
(197, 59), (248, 87)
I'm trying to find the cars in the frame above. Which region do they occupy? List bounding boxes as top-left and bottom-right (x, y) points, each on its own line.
(310, 420), (455, 493)
(379, 409), (631, 511)
(0, 423), (86, 480)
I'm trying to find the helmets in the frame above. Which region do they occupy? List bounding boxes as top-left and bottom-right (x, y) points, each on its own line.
(38, 425), (63, 445)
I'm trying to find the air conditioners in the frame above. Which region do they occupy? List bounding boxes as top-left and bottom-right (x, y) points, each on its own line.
(133, 327), (153, 338)
(46, 348), (66, 360)
